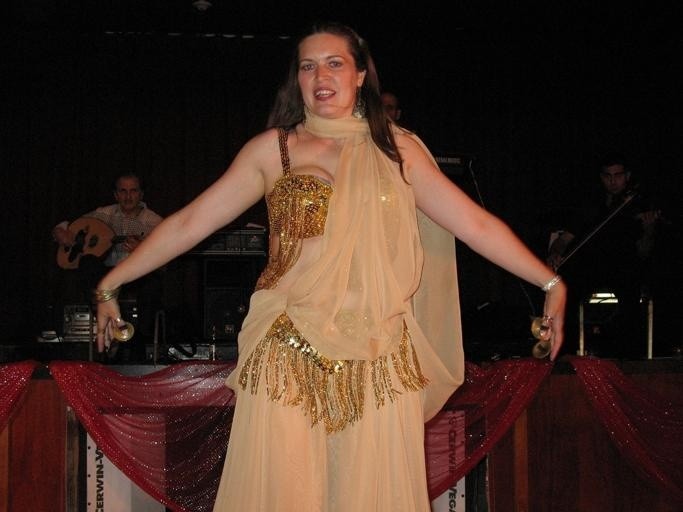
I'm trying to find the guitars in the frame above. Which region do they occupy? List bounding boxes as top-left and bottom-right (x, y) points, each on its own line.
(56, 218), (144, 269)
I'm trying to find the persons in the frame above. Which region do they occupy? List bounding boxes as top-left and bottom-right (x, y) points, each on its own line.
(557, 156), (660, 359)
(382, 90), (401, 126)
(47, 171), (164, 366)
(94, 24), (570, 512)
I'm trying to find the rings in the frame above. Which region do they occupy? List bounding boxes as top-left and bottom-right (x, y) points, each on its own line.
(544, 315), (553, 323)
(113, 318), (122, 323)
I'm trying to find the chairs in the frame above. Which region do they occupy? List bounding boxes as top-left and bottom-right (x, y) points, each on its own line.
(578, 296), (656, 362)
(87, 293), (167, 369)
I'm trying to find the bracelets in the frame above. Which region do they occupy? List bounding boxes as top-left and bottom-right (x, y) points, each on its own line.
(95, 286), (120, 304)
(541, 274), (560, 292)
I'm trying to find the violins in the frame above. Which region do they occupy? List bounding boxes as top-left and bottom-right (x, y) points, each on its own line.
(607, 184), (683, 225)
